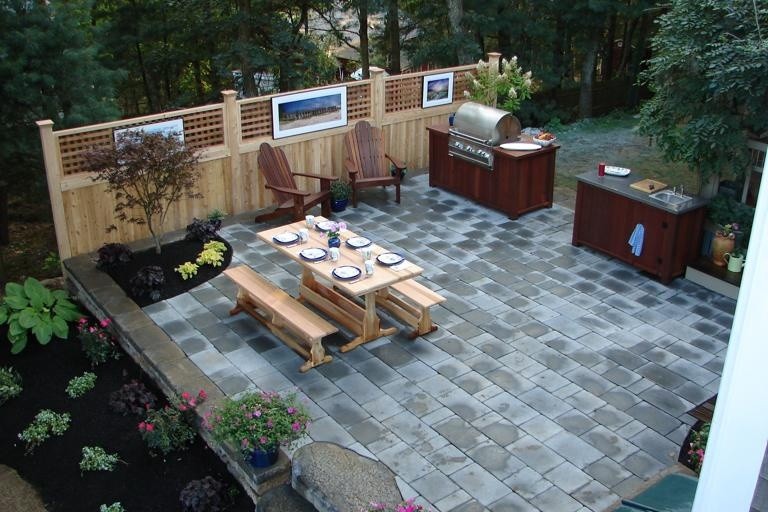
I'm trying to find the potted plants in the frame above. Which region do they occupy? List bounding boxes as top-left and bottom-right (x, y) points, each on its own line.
(719, 222), (738, 235)
(723, 248), (746, 273)
(707, 195), (754, 255)
(330, 180), (352, 212)
(390, 162), (409, 179)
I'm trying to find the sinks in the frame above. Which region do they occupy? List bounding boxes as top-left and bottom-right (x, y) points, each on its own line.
(648, 188), (694, 207)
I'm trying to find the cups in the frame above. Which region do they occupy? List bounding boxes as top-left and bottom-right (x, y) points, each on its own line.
(648, 181), (654, 190)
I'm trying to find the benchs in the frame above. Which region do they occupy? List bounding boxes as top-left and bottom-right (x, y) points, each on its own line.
(222, 265), (339, 372)
(375, 279), (447, 340)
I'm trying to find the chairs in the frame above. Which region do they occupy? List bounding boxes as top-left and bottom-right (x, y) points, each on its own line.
(255, 143), (339, 222)
(344, 120), (407, 207)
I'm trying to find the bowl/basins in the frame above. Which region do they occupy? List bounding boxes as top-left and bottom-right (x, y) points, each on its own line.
(533, 135), (557, 147)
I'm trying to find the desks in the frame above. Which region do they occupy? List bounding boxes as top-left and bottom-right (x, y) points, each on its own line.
(256, 216), (424, 353)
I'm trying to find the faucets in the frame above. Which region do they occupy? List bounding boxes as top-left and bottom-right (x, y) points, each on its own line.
(673, 185), (677, 195)
(680, 184), (685, 196)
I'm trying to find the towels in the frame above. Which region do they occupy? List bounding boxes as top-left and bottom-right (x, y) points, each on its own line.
(628, 223), (645, 257)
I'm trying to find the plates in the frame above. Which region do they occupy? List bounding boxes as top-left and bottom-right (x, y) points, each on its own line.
(499, 142), (542, 151)
(273, 215), (405, 281)
(604, 165), (631, 176)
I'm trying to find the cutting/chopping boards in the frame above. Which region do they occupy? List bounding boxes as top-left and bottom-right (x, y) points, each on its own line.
(629, 179), (668, 194)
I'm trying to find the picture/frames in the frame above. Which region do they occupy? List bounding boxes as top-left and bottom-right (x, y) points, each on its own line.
(112, 117), (185, 166)
(421, 71), (454, 109)
(270, 85), (348, 140)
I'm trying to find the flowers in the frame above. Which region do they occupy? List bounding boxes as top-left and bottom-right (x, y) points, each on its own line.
(201, 386), (312, 460)
(320, 221), (347, 238)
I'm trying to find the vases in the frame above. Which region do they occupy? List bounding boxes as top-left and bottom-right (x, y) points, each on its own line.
(328, 238), (340, 248)
(710, 231), (735, 267)
(242, 442), (279, 467)
